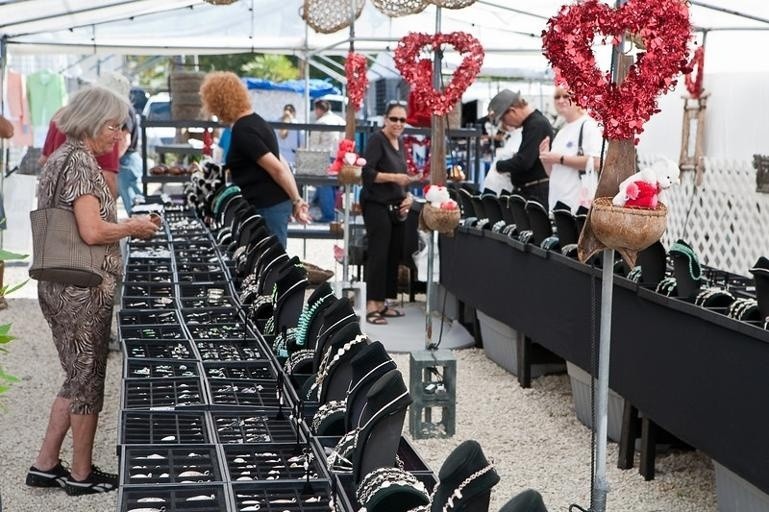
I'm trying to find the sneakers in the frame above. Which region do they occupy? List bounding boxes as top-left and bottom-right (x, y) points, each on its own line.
(65, 465), (119, 495)
(26, 459), (72, 488)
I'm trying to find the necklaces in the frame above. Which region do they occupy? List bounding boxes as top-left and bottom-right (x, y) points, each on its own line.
(456, 183), (586, 255)
(613, 244), (769, 331)
(186, 171), (494, 511)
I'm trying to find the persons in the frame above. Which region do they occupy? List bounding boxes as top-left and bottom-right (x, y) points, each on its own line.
(198, 69), (309, 253)
(311, 99), (347, 223)
(359, 104), (415, 327)
(537, 83), (610, 232)
(0, 112), (13, 311)
(475, 90), (554, 212)
(24, 85), (157, 495)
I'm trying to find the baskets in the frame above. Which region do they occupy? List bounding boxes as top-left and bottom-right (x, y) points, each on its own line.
(338, 165), (363, 184)
(423, 203), (461, 230)
(591, 197), (667, 272)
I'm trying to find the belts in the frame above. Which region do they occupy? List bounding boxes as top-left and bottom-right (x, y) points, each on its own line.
(517, 178), (549, 192)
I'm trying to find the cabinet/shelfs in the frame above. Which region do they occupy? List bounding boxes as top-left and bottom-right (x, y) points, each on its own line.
(140, 115), (483, 239)
(333, 184), (415, 302)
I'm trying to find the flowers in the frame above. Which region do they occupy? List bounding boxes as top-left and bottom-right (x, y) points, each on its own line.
(344, 52), (369, 113)
(540, 0), (694, 146)
(684, 46), (704, 100)
(393, 31), (485, 116)
(402, 135), (432, 183)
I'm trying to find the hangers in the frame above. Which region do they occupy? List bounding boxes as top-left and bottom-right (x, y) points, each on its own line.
(40, 68), (51, 76)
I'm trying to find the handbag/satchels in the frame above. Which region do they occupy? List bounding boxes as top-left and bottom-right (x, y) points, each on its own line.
(384, 200), (408, 224)
(29, 208), (106, 288)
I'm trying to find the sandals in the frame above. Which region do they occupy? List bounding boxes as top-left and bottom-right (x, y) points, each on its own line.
(380, 306), (405, 317)
(367, 311), (387, 324)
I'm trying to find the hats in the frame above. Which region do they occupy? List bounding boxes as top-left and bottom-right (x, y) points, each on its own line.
(488, 89), (520, 124)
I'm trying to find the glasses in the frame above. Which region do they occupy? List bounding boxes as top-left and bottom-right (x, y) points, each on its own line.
(384, 100), (408, 114)
(554, 95), (569, 99)
(103, 124), (124, 133)
(389, 117), (406, 123)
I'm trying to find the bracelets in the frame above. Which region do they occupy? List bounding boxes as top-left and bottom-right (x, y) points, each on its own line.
(560, 155), (564, 165)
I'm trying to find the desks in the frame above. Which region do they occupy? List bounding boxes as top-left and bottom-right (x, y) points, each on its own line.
(115, 209), (441, 512)
(438, 225), (769, 495)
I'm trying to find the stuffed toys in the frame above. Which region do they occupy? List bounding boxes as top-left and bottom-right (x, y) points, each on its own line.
(328, 140), (366, 176)
(611, 159), (681, 209)
